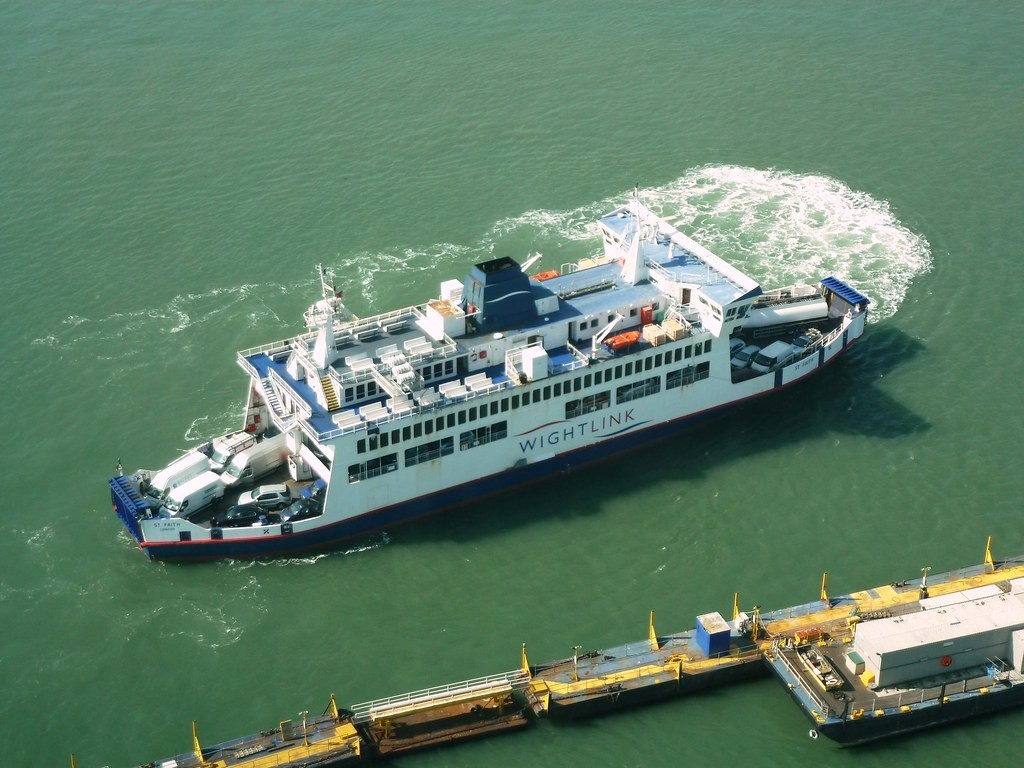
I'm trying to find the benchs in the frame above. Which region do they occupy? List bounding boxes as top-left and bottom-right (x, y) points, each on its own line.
(265, 317), (495, 426)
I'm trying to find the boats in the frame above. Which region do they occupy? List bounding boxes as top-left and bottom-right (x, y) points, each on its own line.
(530, 270), (559, 281)
(95, 201), (868, 553)
(603, 330), (640, 349)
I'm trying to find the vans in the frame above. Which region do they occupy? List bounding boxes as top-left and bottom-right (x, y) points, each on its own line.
(208, 432), (257, 473)
(752, 340), (795, 376)
(149, 449), (212, 503)
(220, 432), (291, 487)
(160, 471), (225, 521)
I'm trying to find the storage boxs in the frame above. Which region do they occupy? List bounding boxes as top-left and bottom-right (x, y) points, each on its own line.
(643, 325), (665, 346)
(662, 319), (685, 341)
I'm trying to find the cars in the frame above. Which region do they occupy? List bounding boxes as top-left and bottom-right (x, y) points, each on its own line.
(210, 503), (271, 526)
(790, 331), (823, 357)
(302, 478), (327, 498)
(277, 498), (322, 523)
(731, 345), (761, 371)
(238, 484), (293, 511)
(730, 337), (745, 354)
